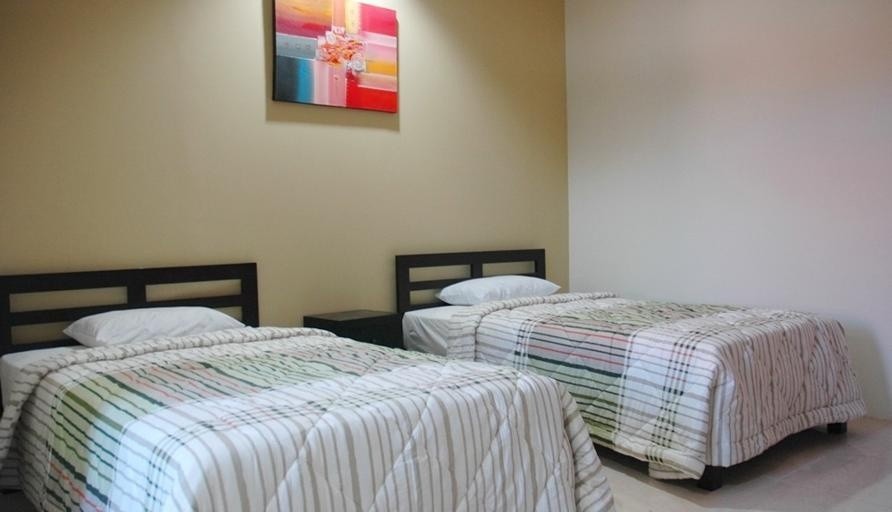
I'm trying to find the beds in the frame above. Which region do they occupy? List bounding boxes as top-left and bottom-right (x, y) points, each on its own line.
(1, 260), (617, 511)
(395, 244), (866, 494)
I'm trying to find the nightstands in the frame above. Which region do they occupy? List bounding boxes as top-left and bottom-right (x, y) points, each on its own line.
(300, 307), (405, 355)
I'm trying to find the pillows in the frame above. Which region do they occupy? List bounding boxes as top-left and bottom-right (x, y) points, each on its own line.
(430, 272), (564, 311)
(61, 304), (250, 350)
